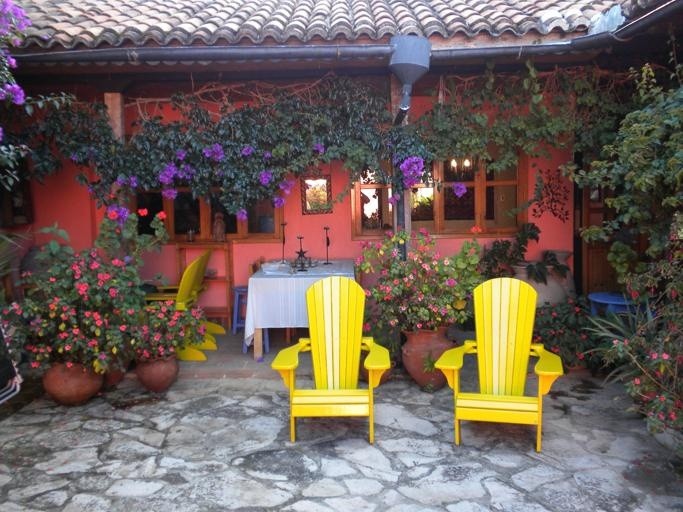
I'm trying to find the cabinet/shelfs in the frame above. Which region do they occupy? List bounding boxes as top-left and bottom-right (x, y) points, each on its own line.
(174, 239), (235, 333)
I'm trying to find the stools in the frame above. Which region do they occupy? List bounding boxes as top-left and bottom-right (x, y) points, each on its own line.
(232, 286), (248, 335)
(587, 291), (656, 320)
(242, 328), (270, 354)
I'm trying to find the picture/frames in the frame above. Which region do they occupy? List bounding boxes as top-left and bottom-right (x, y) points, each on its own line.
(300, 174), (334, 215)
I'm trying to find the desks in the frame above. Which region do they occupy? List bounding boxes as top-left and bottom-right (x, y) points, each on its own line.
(244, 258), (355, 360)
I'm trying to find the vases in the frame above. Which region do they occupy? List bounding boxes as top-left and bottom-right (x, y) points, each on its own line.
(38, 341), (179, 407)
(399, 323), (457, 393)
(362, 362), (396, 386)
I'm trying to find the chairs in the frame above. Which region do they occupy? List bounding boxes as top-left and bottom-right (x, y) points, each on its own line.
(434, 275), (563, 453)
(143, 248), (225, 361)
(273, 275), (389, 445)
(247, 256), (298, 345)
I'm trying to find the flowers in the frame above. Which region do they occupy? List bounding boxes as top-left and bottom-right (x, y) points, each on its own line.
(354, 225), (486, 359)
(0, 189), (207, 376)
